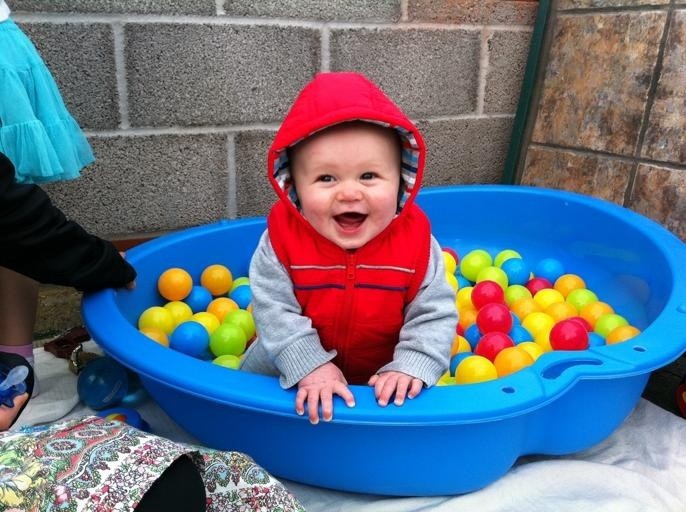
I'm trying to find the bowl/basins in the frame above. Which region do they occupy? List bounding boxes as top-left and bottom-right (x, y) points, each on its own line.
(80, 183), (686, 494)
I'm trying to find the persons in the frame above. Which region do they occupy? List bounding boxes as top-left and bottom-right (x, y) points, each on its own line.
(238, 70), (459, 424)
(0, 151), (303, 508)
(0, 0), (95, 397)
